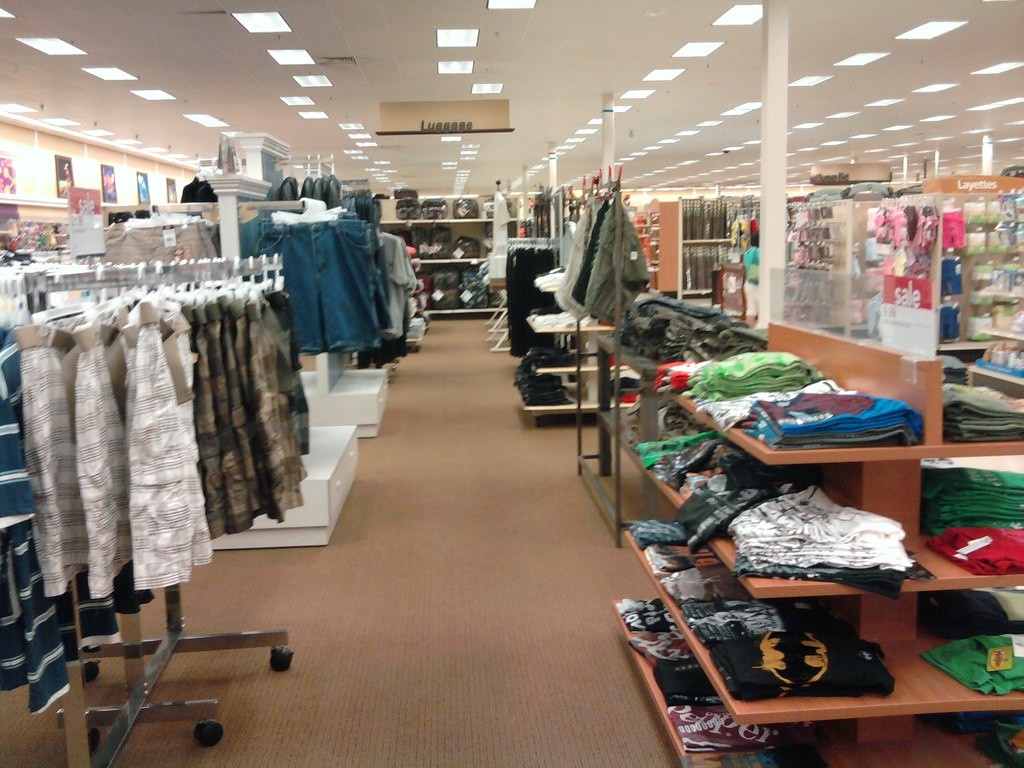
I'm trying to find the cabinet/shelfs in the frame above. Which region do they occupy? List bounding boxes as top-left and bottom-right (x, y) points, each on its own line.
(613, 319), (1024, 768)
(378, 217), (522, 313)
(631, 195), (760, 298)
(521, 273), (633, 417)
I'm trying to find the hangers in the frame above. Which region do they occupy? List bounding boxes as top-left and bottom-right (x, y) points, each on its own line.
(123, 199), (215, 232)
(270, 197), (347, 228)
(506, 236), (560, 253)
(0, 249), (285, 331)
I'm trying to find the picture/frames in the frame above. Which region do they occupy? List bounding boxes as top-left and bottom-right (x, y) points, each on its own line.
(56, 154), (75, 198)
(136, 170), (151, 204)
(101, 163), (118, 203)
(166, 177), (178, 203)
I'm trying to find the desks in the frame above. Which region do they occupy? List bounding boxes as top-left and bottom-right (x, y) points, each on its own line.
(596, 334), (669, 523)
